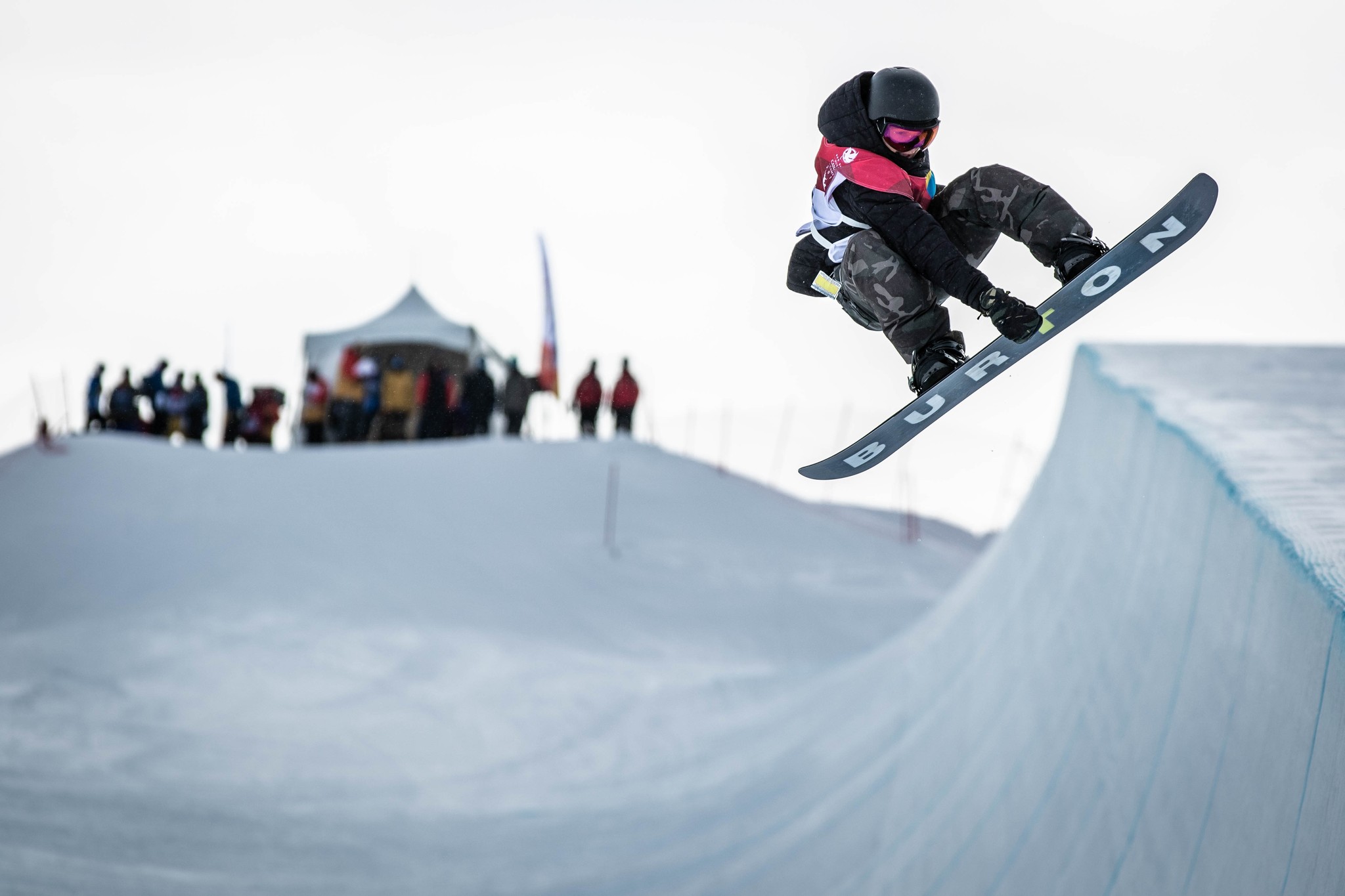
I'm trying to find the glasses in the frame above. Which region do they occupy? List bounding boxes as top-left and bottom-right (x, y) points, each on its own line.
(880, 120), (941, 153)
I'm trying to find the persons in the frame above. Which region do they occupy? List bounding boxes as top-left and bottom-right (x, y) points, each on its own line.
(302, 370), (329, 443)
(167, 374), (190, 435)
(461, 357), (495, 435)
(415, 356), (456, 437)
(572, 361), (602, 436)
(217, 372), (241, 443)
(611, 359), (638, 434)
(186, 375), (207, 439)
(110, 370), (138, 431)
(379, 355), (413, 440)
(785, 67), (1106, 385)
(330, 349), (378, 441)
(147, 362), (168, 391)
(504, 358), (530, 434)
(86, 365), (106, 430)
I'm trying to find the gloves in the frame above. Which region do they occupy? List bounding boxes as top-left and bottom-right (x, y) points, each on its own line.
(977, 287), (1043, 344)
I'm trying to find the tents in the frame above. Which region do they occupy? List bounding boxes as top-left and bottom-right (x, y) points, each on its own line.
(299, 287), (481, 444)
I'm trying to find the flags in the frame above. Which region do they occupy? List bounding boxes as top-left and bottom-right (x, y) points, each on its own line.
(537, 236), (556, 393)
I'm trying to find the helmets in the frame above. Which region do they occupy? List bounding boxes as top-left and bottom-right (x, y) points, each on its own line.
(867, 66), (939, 120)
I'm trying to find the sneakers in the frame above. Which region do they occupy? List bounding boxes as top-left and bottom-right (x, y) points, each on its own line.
(1054, 232), (1101, 285)
(912, 342), (963, 396)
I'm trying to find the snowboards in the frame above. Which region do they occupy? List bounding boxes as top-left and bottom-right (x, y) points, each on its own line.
(797, 172), (1219, 480)
